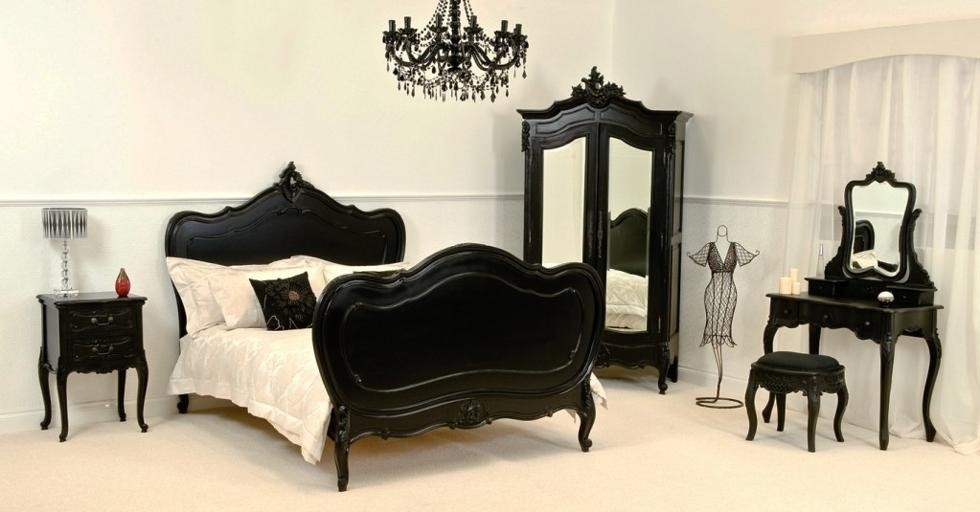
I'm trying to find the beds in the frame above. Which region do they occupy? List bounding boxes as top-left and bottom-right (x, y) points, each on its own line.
(165, 160), (610, 491)
(605, 207), (650, 332)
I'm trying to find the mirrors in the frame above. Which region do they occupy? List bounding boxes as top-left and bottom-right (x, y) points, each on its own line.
(538, 134), (586, 269)
(826, 161), (936, 289)
(605, 136), (654, 334)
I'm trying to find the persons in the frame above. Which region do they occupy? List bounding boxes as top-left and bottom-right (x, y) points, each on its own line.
(684, 225), (761, 348)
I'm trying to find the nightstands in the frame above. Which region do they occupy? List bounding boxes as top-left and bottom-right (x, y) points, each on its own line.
(36, 290), (150, 441)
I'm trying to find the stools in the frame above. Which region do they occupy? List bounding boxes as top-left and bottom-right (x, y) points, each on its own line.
(744, 351), (851, 453)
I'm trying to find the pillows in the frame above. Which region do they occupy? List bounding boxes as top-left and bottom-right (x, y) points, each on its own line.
(204, 263), (328, 330)
(292, 253), (416, 288)
(166, 253), (327, 337)
(250, 271), (316, 331)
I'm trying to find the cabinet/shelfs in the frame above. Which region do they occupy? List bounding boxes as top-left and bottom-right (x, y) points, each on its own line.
(516, 66), (693, 395)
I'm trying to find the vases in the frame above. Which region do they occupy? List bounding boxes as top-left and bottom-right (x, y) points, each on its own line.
(116, 267), (131, 298)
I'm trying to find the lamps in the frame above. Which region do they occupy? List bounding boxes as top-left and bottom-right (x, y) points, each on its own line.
(382, 0), (529, 104)
(41, 206), (88, 296)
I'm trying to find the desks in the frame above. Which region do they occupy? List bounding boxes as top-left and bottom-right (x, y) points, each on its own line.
(761, 290), (945, 451)
